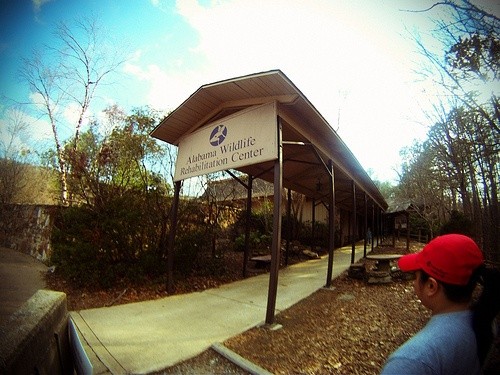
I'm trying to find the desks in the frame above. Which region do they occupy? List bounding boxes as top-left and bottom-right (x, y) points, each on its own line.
(366, 254), (402, 269)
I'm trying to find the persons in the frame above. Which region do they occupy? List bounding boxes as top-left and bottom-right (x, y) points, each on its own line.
(377, 233), (500, 375)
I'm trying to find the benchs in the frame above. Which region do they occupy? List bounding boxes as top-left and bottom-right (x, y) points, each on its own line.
(251, 255), (271, 273)
(349, 264), (364, 280)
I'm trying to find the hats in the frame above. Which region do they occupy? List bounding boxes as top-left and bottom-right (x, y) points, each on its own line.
(398, 234), (484, 284)
